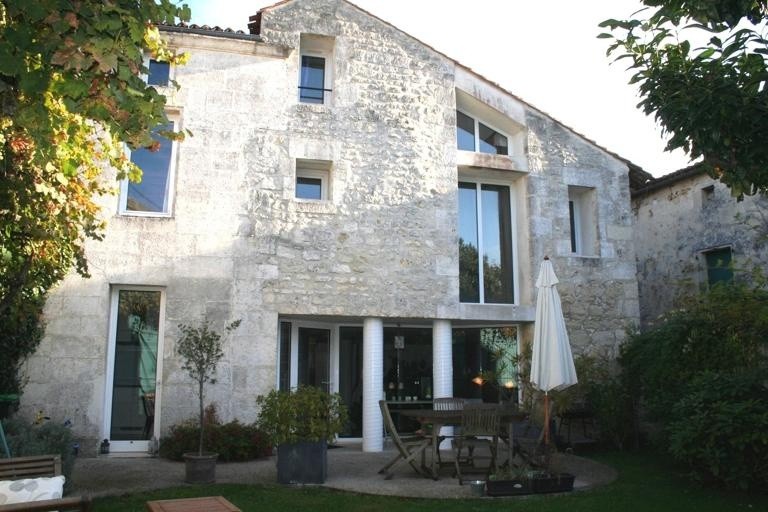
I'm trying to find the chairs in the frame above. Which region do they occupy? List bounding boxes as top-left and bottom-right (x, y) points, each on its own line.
(376, 399), (445, 482)
(449, 402), (502, 485)
(431, 397), (477, 468)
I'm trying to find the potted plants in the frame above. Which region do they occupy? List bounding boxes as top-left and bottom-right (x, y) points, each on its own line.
(487, 464), (529, 495)
(532, 462), (578, 493)
(255, 383), (352, 486)
(171, 310), (243, 487)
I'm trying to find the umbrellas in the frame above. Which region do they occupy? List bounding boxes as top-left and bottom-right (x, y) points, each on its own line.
(529, 256), (579, 443)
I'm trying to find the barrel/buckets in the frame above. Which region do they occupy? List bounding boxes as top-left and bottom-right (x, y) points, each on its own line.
(470, 480), (486, 497)
(404, 442), (426, 474)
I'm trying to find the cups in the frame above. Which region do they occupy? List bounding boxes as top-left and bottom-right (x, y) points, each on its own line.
(413, 396), (418, 400)
(405, 396), (411, 401)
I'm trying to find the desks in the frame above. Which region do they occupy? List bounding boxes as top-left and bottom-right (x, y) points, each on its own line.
(401, 408), (529, 478)
